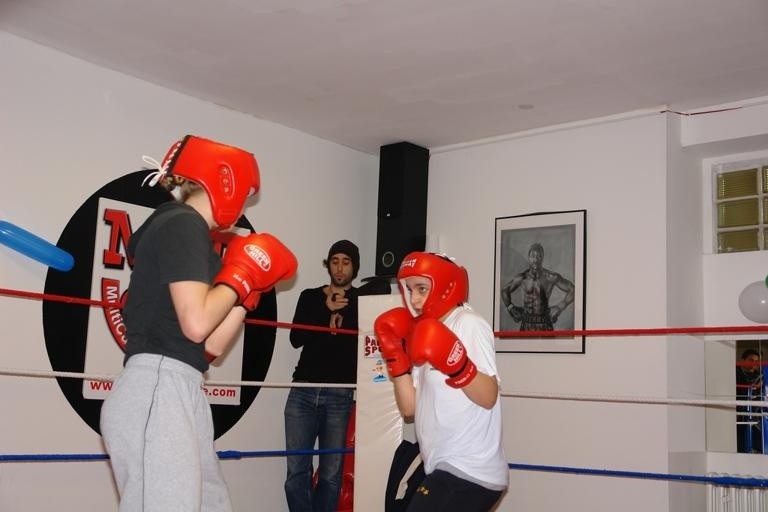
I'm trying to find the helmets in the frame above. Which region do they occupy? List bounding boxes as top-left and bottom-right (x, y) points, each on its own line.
(154, 134), (260, 232)
(397, 251), (469, 323)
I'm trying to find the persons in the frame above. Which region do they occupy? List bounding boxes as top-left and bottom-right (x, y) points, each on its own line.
(501, 243), (575, 331)
(99, 135), (298, 512)
(736, 350), (763, 453)
(374, 251), (510, 512)
(285, 240), (391, 512)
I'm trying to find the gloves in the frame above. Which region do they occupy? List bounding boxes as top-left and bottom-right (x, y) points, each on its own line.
(507, 304), (523, 323)
(374, 306), (477, 388)
(550, 305), (561, 323)
(213, 233), (298, 311)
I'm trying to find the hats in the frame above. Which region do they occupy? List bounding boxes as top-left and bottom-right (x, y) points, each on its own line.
(329, 240), (359, 277)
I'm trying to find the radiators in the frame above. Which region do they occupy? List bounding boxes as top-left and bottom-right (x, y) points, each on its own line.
(704, 472), (768, 510)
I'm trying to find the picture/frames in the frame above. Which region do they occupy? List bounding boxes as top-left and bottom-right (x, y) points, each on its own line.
(492, 208), (589, 355)
